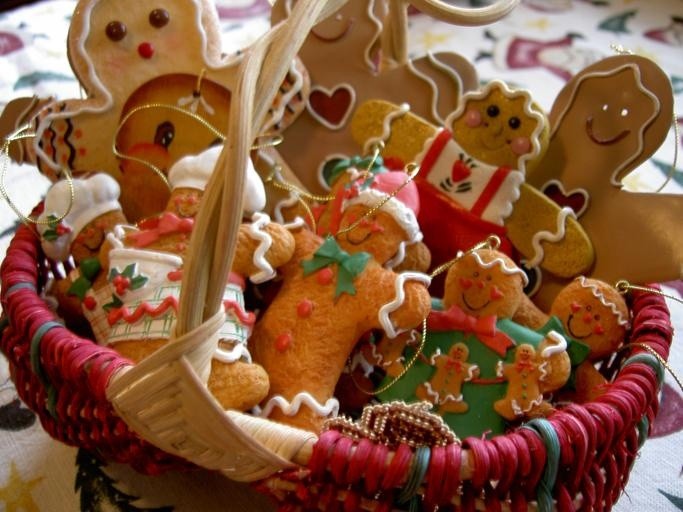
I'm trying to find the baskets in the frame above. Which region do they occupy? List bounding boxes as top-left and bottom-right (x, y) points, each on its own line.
(0, 1), (676, 509)
